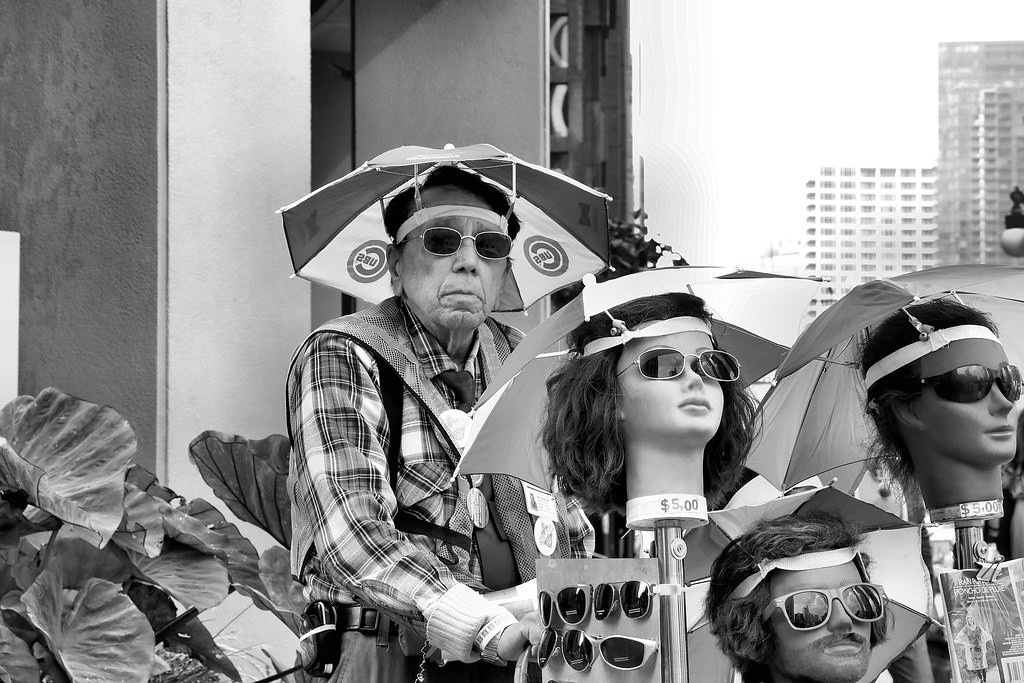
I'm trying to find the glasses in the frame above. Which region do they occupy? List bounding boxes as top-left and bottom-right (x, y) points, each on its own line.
(615, 348), (741, 383)
(402, 226), (514, 261)
(593, 579), (650, 621)
(920, 364), (1024, 404)
(537, 627), (599, 673)
(762, 583), (890, 631)
(537, 585), (591, 628)
(598, 634), (658, 671)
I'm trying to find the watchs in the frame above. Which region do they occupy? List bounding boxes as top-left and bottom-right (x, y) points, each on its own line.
(480, 628), (508, 667)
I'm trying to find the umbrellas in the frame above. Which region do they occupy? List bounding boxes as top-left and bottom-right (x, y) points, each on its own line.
(684, 478), (945, 683)
(275, 143), (618, 318)
(741, 264), (1024, 498)
(449, 267), (832, 497)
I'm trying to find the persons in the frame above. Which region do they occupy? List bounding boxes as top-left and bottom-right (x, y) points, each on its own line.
(283, 166), (596, 683)
(860, 299), (1024, 524)
(543, 294), (764, 530)
(706, 512), (889, 683)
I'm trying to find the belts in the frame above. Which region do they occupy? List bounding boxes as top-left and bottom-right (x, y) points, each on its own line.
(341, 606), (400, 637)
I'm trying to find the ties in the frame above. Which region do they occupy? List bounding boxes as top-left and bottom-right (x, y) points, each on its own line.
(437, 369), (521, 591)
